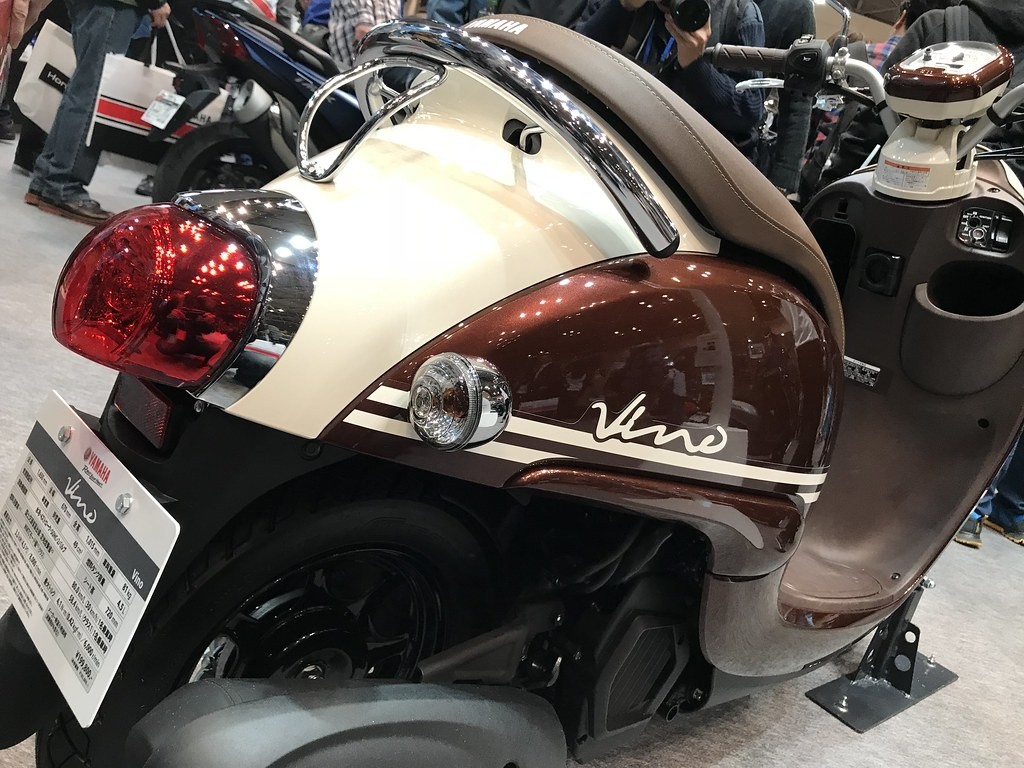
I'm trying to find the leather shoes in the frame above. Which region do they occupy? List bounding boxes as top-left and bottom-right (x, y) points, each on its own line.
(23, 187), (116, 227)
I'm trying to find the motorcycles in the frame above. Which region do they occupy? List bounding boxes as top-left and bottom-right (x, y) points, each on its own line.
(4, 2), (1024, 766)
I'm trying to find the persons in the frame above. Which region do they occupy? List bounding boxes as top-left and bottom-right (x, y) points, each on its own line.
(744, 0), (817, 209)
(571, 0), (774, 178)
(328, 1), (426, 110)
(848, 0), (1024, 208)
(809, 0), (947, 194)
(24, 0), (174, 226)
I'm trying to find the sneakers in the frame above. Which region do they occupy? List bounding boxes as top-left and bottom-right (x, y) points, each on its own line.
(984, 514), (1024, 544)
(136, 173), (155, 196)
(953, 511), (984, 549)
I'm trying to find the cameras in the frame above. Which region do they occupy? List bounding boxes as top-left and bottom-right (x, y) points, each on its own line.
(650, 0), (710, 32)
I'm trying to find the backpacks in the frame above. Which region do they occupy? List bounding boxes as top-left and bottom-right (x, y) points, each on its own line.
(945, 4), (1024, 185)
